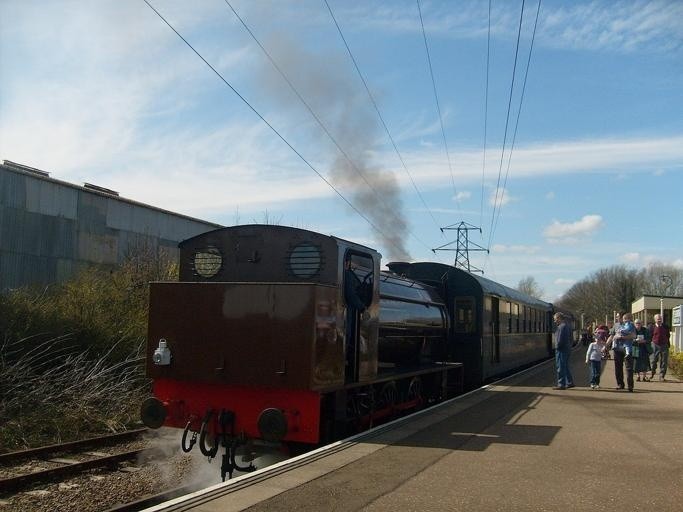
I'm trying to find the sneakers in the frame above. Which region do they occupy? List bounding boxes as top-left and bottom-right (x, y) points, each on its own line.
(636, 372), (665, 382)
(553, 387), (564, 390)
(628, 386), (633, 392)
(566, 384), (575, 388)
(617, 384), (624, 388)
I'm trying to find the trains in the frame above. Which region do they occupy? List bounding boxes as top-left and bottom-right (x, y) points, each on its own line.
(139, 224), (581, 485)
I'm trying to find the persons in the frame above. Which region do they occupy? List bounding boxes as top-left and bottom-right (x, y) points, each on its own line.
(553, 312), (578, 390)
(583, 311), (671, 393)
